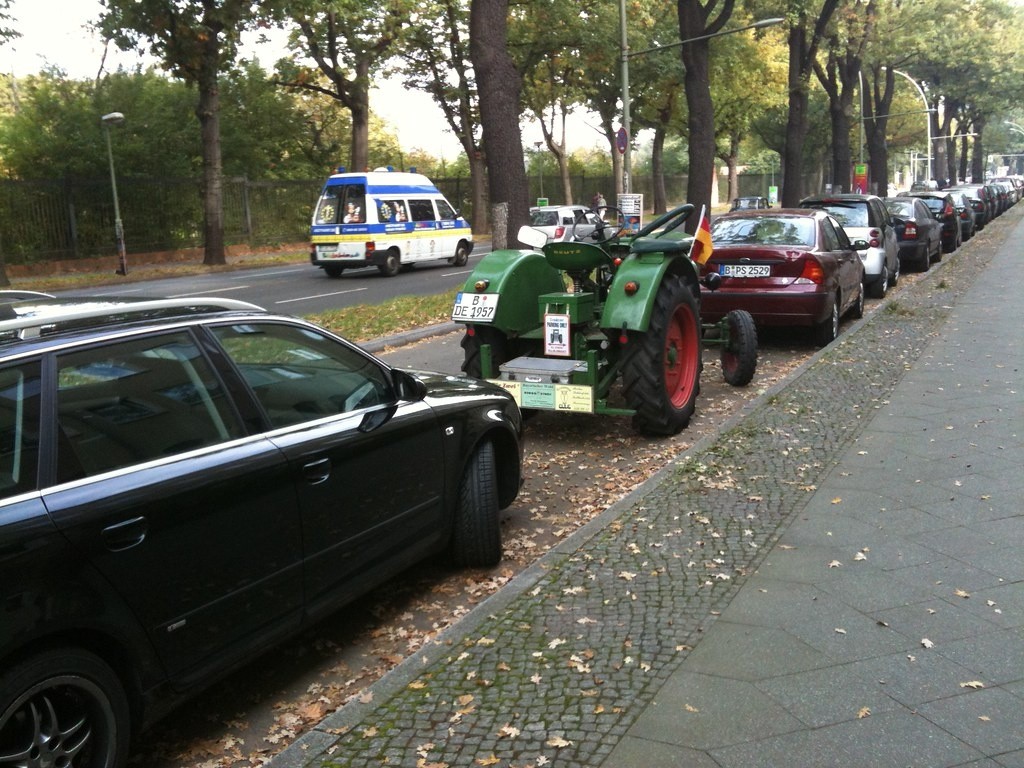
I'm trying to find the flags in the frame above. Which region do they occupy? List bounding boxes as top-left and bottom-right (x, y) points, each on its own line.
(689, 209), (714, 266)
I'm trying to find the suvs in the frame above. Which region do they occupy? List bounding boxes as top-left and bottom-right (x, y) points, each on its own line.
(0, 288), (526, 768)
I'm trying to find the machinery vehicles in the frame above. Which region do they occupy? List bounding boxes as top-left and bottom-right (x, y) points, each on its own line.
(447, 204), (762, 438)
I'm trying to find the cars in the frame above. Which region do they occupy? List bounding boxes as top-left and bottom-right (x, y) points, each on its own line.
(793, 192), (907, 299)
(877, 194), (944, 273)
(727, 195), (773, 214)
(688, 207), (870, 347)
(896, 191), (963, 254)
(939, 172), (1024, 240)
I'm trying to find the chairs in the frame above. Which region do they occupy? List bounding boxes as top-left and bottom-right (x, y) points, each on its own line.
(542, 240), (613, 275)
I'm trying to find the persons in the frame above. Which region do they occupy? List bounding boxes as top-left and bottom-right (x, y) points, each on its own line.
(591, 192), (607, 220)
(938, 175), (947, 191)
(929, 177), (938, 191)
(855, 183), (862, 194)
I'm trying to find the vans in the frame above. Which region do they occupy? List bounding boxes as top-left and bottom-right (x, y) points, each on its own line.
(529, 204), (616, 250)
(307, 163), (476, 279)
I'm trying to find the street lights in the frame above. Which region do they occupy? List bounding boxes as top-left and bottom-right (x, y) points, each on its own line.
(804, 11), (937, 193)
(101, 111), (130, 277)
(617, 16), (786, 192)
(882, 65), (980, 190)
